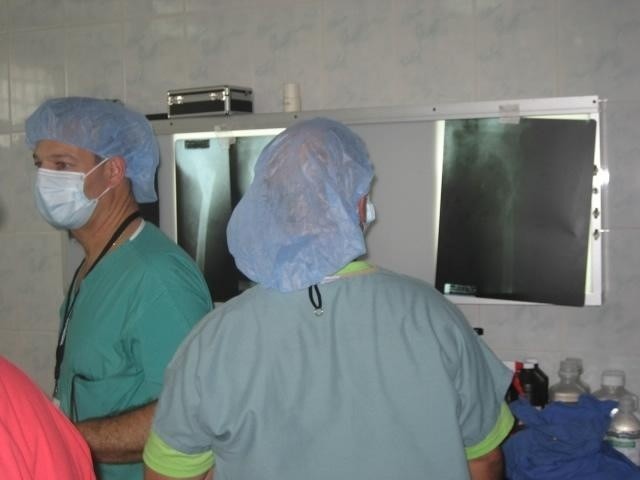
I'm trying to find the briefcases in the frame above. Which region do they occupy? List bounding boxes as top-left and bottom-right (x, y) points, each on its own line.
(165, 85), (254, 120)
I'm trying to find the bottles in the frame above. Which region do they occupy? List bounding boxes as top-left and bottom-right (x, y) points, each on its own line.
(282, 81), (301, 113)
(510, 357), (639, 411)
(604, 396), (639, 467)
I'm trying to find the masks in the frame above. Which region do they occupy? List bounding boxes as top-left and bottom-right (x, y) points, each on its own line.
(31, 157), (114, 232)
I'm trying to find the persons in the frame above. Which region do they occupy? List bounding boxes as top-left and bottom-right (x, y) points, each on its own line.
(139, 114), (517, 480)
(19, 95), (210, 479)
